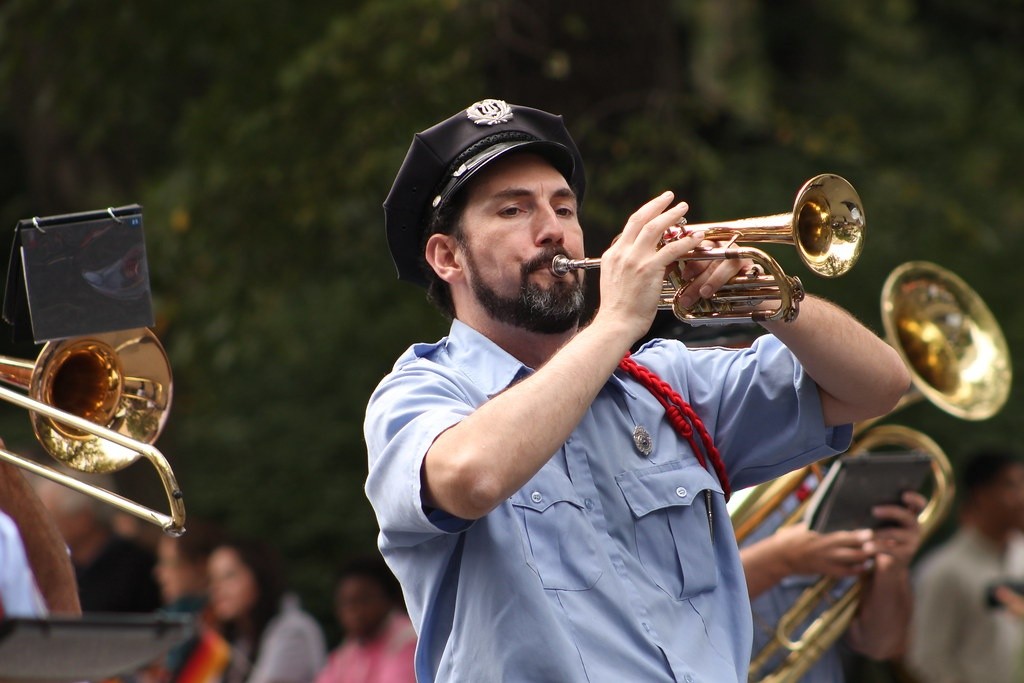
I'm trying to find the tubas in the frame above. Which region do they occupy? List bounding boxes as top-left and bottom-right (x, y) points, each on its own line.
(725, 262), (1015, 683)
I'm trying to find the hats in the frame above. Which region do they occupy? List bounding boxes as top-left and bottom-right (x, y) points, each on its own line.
(382, 100), (587, 301)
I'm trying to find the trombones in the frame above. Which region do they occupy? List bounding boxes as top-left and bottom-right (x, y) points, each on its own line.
(0, 327), (188, 536)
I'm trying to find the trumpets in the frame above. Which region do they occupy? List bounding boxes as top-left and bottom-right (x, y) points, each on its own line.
(548, 172), (867, 329)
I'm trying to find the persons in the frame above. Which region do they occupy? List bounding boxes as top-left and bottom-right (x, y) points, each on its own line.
(895, 443), (1024, 683)
(0, 437), (83, 618)
(725, 469), (917, 683)
(364, 99), (909, 683)
(13, 449), (418, 683)
